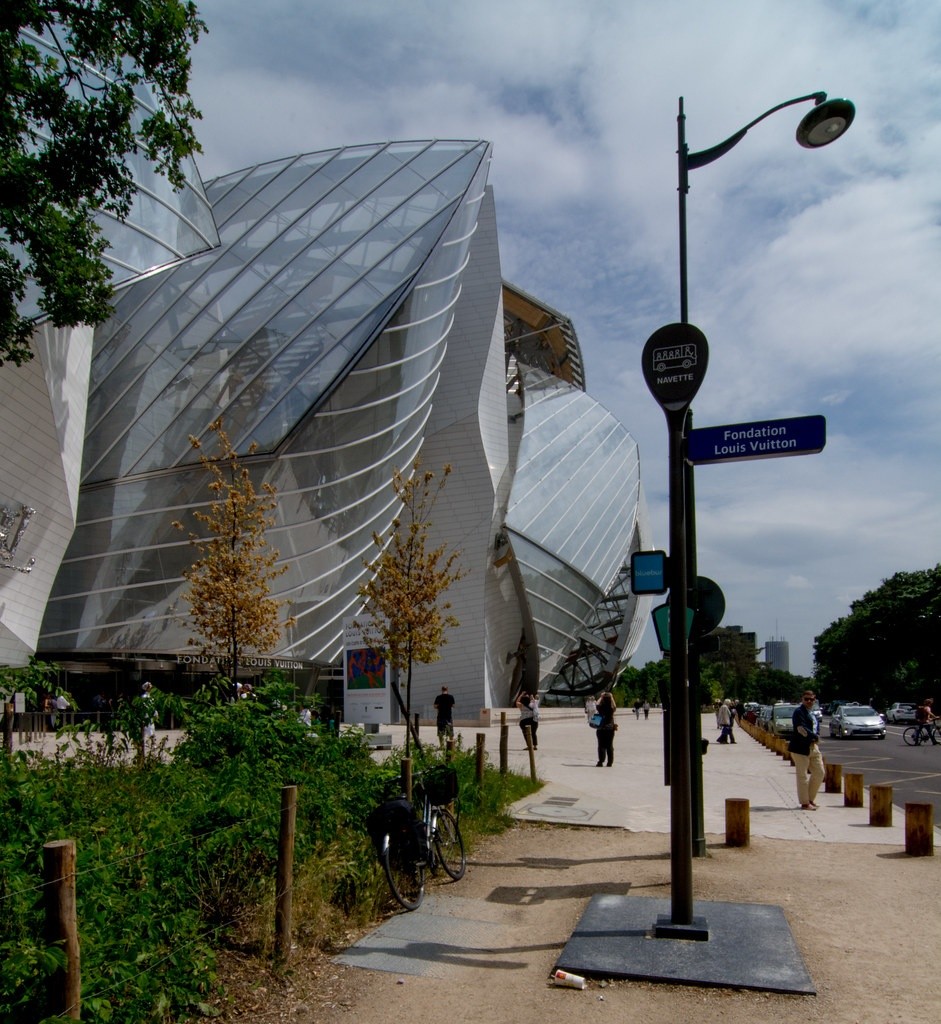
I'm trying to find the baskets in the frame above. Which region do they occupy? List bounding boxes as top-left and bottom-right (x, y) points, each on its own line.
(414, 765), (459, 806)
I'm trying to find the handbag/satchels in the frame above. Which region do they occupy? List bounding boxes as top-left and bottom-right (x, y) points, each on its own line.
(589, 713), (606, 729)
(366, 800), (428, 870)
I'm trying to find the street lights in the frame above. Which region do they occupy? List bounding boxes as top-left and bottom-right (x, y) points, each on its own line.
(675, 93), (855, 860)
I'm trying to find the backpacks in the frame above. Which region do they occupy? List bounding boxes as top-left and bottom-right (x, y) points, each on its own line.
(916, 706), (928, 719)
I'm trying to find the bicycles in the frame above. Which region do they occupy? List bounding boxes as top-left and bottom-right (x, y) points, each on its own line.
(903, 719), (941, 746)
(378, 764), (464, 911)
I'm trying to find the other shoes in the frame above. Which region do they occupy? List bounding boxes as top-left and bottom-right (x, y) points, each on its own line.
(596, 764), (603, 767)
(809, 800), (819, 807)
(607, 764), (612, 767)
(802, 804), (817, 810)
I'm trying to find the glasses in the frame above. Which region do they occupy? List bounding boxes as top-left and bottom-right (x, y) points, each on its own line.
(803, 698), (815, 701)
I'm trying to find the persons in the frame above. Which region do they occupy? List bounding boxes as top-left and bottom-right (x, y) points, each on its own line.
(226, 682), (334, 735)
(140, 681), (158, 748)
(434, 686), (455, 747)
(634, 698), (641, 719)
(595, 692), (617, 767)
(910, 697), (940, 746)
(643, 700), (649, 719)
(713, 698), (744, 745)
(518, 691), (540, 750)
(871, 696), (881, 713)
(584, 696), (597, 722)
(43, 692), (71, 729)
(787, 690), (826, 811)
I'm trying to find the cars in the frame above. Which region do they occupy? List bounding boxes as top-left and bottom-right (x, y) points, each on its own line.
(741, 700), (823, 739)
(829, 702), (886, 740)
(822, 701), (920, 725)
